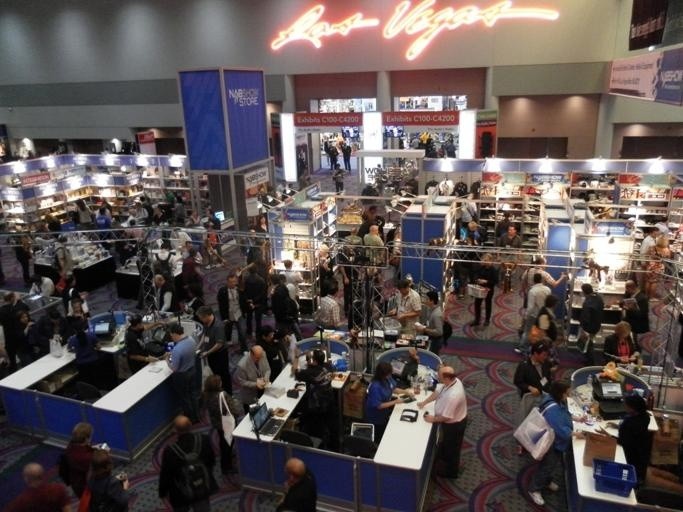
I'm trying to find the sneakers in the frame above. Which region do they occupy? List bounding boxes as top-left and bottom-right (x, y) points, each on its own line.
(529, 490), (543, 505)
(538, 480), (558, 490)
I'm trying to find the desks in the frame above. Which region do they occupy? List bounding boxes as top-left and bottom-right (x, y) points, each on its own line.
(0, 329), (181, 464)
(232, 363), (443, 512)
(565, 382), (658, 512)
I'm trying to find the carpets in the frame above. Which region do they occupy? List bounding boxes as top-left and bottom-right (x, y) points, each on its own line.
(0, 253), (673, 512)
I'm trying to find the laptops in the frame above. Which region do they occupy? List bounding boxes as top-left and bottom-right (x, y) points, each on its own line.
(254, 403), (282, 436)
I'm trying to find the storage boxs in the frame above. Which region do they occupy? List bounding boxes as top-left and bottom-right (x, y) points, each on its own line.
(41, 374), (63, 393)
(343, 372), (373, 418)
(593, 458), (637, 497)
(583, 433), (617, 468)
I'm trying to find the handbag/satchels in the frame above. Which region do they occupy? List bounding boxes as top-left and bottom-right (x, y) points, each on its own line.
(219, 391), (235, 447)
(582, 433), (623, 467)
(514, 403), (558, 461)
(49, 336), (63, 357)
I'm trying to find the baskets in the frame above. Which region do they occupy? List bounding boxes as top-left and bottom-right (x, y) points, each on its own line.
(468, 281), (490, 298)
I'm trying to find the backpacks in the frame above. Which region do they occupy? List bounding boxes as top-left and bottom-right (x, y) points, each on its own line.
(442, 320), (452, 348)
(167, 433), (219, 502)
(154, 251), (175, 281)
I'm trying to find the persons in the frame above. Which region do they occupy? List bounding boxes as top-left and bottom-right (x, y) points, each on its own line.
(514, 256), (569, 340)
(61, 275), (84, 310)
(577, 283), (604, 367)
(185, 284), (204, 319)
(136, 247), (155, 310)
(604, 321), (640, 364)
(183, 246), (204, 292)
(594, 395), (649, 496)
(640, 215), (675, 266)
(399, 99), (431, 111)
(523, 339), (560, 380)
(340, 243), (353, 312)
(275, 458), (317, 512)
(203, 209), (224, 269)
(416, 291), (442, 356)
(158, 415), (216, 511)
(28, 276), (55, 300)
(395, 130), (458, 160)
(513, 342), (552, 457)
(235, 345), (271, 415)
(262, 167), (313, 205)
(318, 244), (333, 295)
(59, 423), (110, 496)
(152, 243), (177, 294)
(318, 284), (345, 330)
(12, 309), (42, 364)
(4, 462), (73, 512)
(79, 451), (132, 512)
(417, 367), (468, 478)
(5, 225), (36, 286)
(364, 362), (415, 451)
(391, 280), (422, 324)
(71, 187), (203, 253)
(44, 214), (62, 236)
(168, 324), (197, 420)
(124, 314), (168, 375)
(270, 284), (299, 342)
(618, 280), (649, 354)
(49, 236), (75, 302)
(295, 147), (308, 172)
(204, 376), (240, 473)
(69, 301), (90, 330)
(360, 163), (419, 265)
(241, 265), (268, 336)
(319, 128), (355, 202)
(470, 254), (497, 327)
(68, 316), (103, 389)
(495, 211), (522, 294)
(0, 292), (30, 371)
(154, 274), (179, 315)
(444, 192), (491, 256)
(239, 196), (270, 278)
(258, 328), (283, 378)
(195, 305), (233, 395)
(271, 259), (304, 300)
(218, 274), (249, 352)
(526, 380), (589, 506)
(36, 309), (65, 352)
(291, 350), (338, 448)
(181, 241), (201, 261)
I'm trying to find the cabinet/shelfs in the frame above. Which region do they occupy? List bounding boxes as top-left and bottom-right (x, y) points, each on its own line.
(268, 197), (337, 322)
(0, 290), (66, 365)
(538, 202), (572, 318)
(34, 257), (117, 297)
(115, 271), (182, 301)
(422, 171), (683, 253)
(402, 196), (456, 312)
(565, 291), (624, 353)
(1, 154), (212, 232)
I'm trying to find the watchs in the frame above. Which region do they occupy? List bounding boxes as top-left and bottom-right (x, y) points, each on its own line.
(145, 356), (149, 362)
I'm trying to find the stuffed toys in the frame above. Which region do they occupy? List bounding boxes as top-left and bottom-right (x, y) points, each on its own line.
(599, 362), (618, 381)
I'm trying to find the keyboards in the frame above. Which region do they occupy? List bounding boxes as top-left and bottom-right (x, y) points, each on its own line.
(598, 402), (631, 414)
(395, 380), (412, 390)
(146, 341), (165, 357)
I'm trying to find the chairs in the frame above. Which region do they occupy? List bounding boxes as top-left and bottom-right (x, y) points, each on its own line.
(342, 432), (378, 457)
(76, 382), (102, 400)
(641, 440), (682, 496)
(279, 429), (322, 448)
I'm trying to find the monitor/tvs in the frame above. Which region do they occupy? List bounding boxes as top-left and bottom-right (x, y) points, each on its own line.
(213, 211), (227, 223)
(600, 381), (622, 399)
(152, 326), (171, 345)
(387, 357), (418, 382)
(94, 321), (109, 336)
